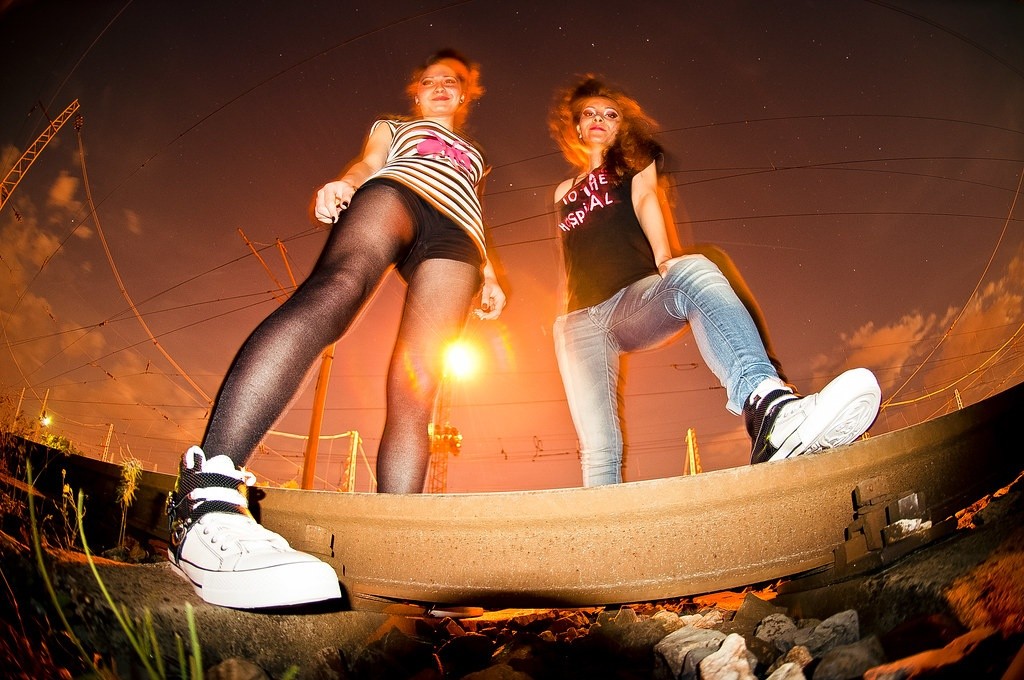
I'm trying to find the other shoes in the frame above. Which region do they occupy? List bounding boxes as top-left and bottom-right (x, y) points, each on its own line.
(165, 446), (343, 608)
(743, 367), (881, 466)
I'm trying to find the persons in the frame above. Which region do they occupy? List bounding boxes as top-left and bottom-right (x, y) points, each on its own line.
(166, 49), (507, 611)
(547, 74), (881, 486)
